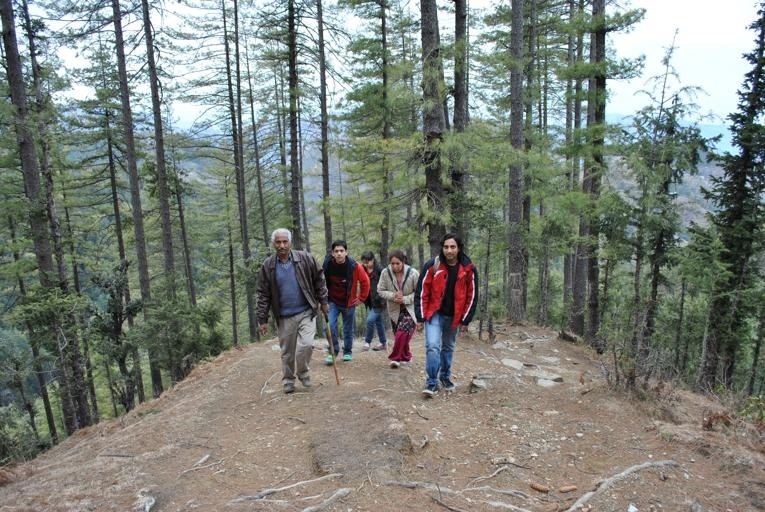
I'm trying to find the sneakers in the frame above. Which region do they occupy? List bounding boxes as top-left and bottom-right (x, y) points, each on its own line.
(372, 342), (388, 352)
(422, 384), (438, 396)
(361, 341), (371, 352)
(342, 351), (352, 362)
(438, 376), (457, 391)
(298, 373), (314, 388)
(282, 377), (295, 394)
(389, 356), (400, 369)
(324, 350), (338, 365)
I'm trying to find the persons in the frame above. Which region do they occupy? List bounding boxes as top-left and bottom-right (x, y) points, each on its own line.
(257, 228), (329, 394)
(376, 251), (420, 369)
(358, 250), (388, 351)
(414, 233), (477, 397)
(322, 239), (371, 365)
